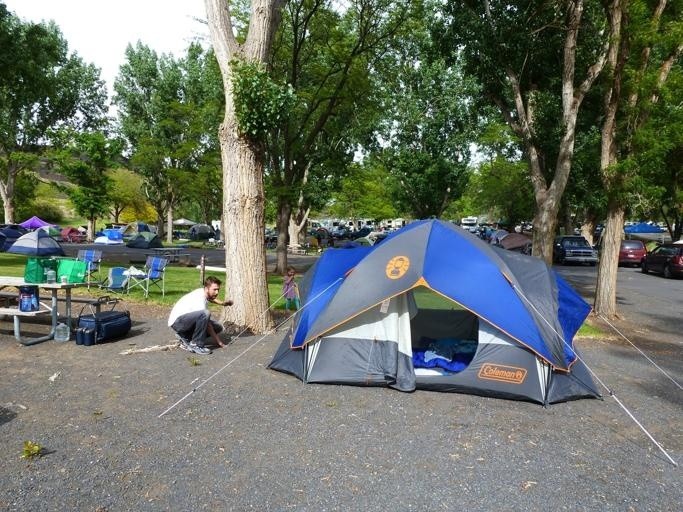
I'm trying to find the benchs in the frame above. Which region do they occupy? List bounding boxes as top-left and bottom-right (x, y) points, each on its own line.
(0, 291), (116, 346)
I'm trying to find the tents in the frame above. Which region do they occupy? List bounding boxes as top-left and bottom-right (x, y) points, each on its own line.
(351, 232), (388, 247)
(1, 216), (88, 256)
(173, 217), (220, 242)
(268, 220), (603, 405)
(486, 228), (531, 250)
(94, 221), (164, 249)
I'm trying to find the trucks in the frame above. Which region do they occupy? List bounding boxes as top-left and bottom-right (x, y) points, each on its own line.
(460, 216), (477, 234)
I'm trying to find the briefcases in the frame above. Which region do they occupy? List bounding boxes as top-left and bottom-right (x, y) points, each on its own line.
(75, 310), (131, 345)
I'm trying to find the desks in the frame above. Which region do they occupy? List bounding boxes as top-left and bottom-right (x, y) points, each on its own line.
(0, 275), (85, 337)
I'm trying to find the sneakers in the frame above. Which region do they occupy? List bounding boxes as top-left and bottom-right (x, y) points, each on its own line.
(180, 341), (212, 355)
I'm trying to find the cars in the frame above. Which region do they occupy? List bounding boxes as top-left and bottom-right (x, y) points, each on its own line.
(618, 240), (682, 280)
(307, 218), (405, 233)
(552, 236), (598, 266)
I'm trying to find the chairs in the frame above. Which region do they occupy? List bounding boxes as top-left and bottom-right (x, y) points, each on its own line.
(74, 249), (169, 299)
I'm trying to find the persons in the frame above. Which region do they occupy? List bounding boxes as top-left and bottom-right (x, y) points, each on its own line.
(316, 231), (333, 252)
(283, 266), (300, 310)
(168, 276), (233, 354)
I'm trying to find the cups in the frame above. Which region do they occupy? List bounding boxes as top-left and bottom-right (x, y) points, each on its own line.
(46, 271), (56, 286)
(60, 276), (68, 286)
(75, 327), (92, 346)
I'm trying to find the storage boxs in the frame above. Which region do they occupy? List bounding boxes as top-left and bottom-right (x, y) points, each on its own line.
(78, 311), (132, 341)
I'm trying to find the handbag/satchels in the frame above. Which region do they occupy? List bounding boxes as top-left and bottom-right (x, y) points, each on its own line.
(55, 258), (87, 283)
(24, 255), (56, 284)
